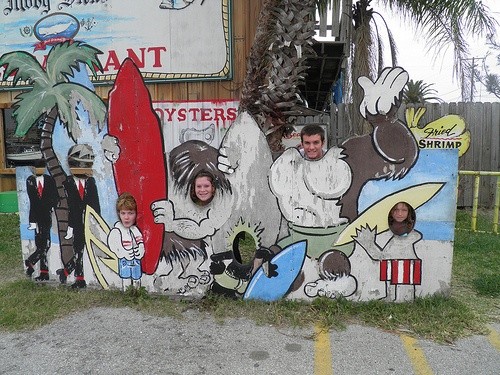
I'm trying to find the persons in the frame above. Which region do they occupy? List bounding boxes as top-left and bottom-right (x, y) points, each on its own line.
(190, 169), (217, 207)
(388, 202), (415, 236)
(116, 192), (138, 229)
(298, 123), (329, 161)
